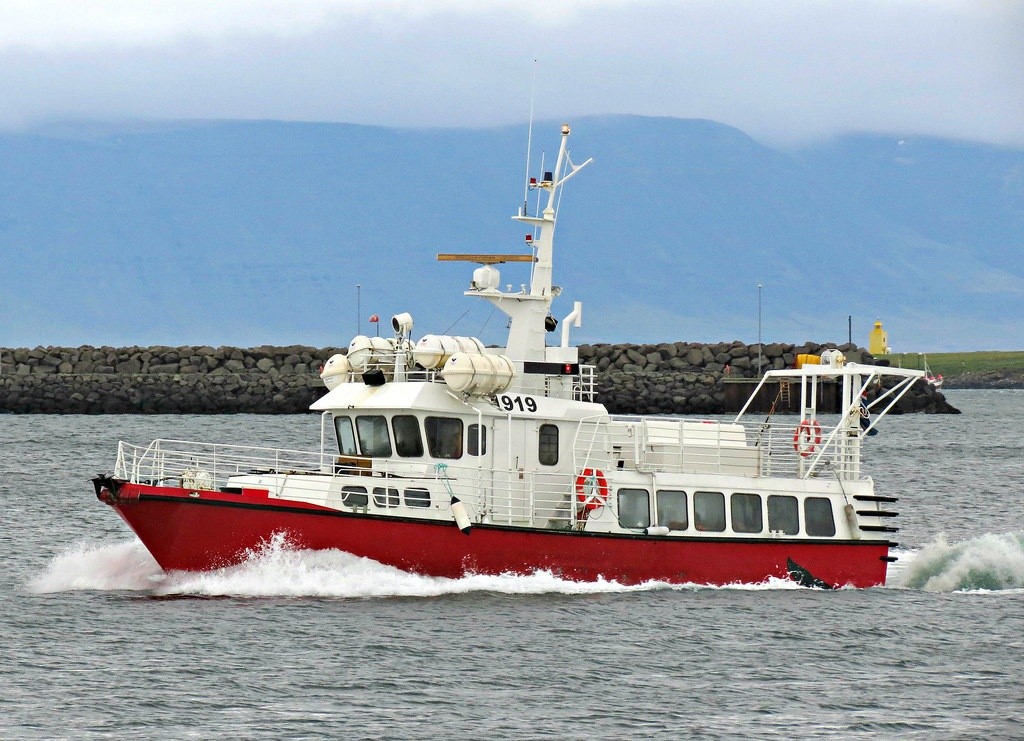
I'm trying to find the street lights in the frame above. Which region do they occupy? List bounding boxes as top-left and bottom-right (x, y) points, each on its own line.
(357, 284), (360, 336)
(758, 284), (761, 374)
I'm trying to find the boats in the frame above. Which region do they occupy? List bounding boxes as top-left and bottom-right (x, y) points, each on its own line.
(89, 106), (925, 599)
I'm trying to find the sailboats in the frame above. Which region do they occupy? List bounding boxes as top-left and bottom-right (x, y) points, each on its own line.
(921, 352), (944, 391)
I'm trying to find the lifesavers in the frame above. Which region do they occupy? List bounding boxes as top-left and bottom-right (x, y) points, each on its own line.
(577, 469), (607, 509)
(793, 419), (821, 457)
